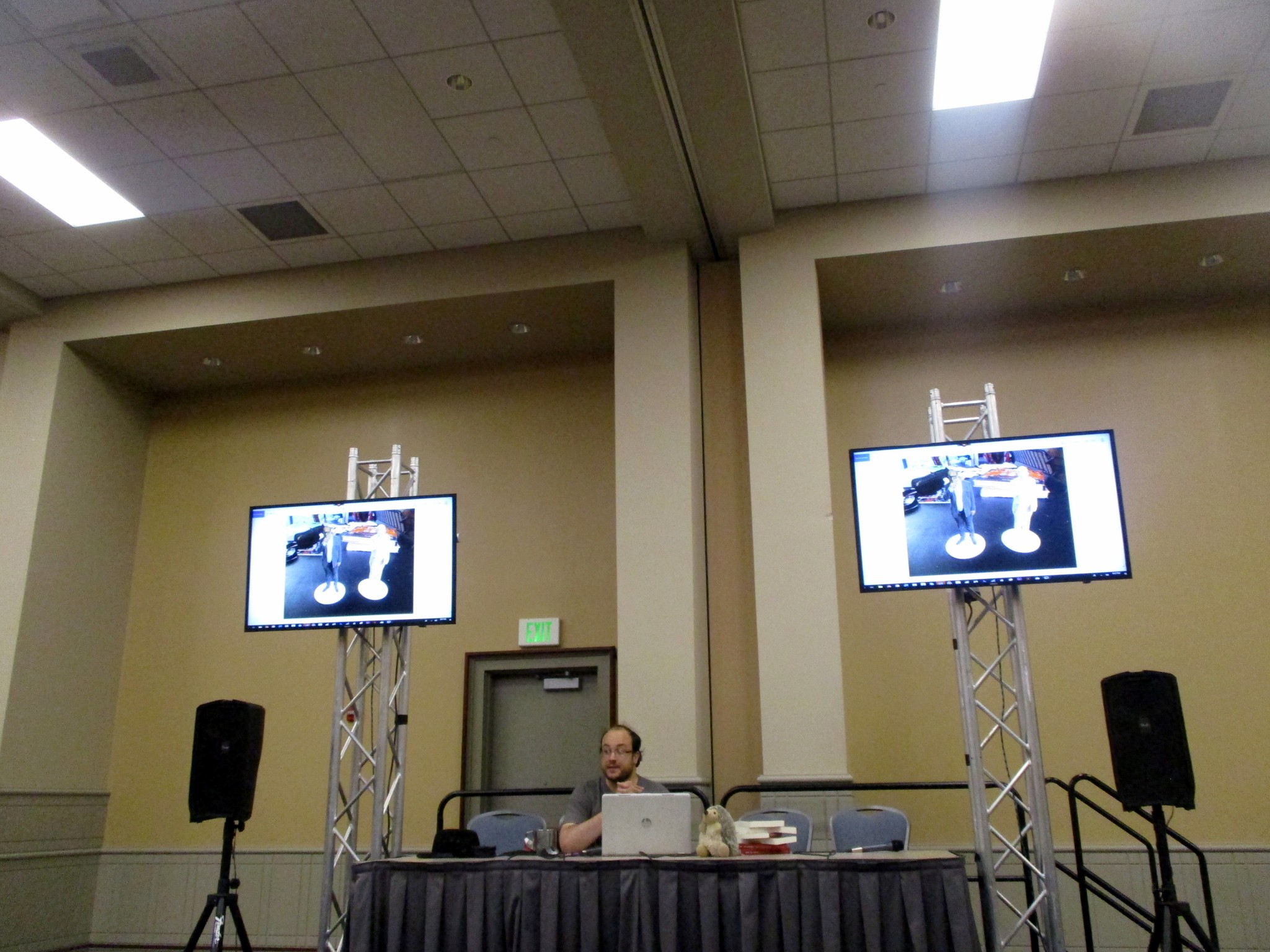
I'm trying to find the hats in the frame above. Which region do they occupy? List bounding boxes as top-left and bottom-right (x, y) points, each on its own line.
(418, 828), (482, 859)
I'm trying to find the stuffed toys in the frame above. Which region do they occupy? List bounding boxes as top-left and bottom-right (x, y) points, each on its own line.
(696, 805), (741, 857)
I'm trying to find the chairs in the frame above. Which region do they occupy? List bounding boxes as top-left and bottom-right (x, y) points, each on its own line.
(738, 808), (813, 853)
(829, 804), (910, 852)
(467, 809), (547, 853)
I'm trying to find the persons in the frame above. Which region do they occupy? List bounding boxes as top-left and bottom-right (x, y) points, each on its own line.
(558, 724), (672, 854)
(320, 526), (342, 592)
(944, 469), (977, 545)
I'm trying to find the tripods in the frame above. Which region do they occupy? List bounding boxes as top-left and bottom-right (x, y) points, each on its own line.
(183, 818), (252, 952)
(1143, 806), (1218, 951)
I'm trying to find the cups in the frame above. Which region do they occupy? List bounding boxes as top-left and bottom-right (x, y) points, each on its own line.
(526, 828), (557, 854)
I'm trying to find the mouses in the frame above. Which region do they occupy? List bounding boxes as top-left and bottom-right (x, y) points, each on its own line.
(544, 846), (560, 857)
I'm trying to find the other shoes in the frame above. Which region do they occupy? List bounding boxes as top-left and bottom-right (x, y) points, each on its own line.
(334, 584), (338, 592)
(323, 586), (330, 592)
(970, 534), (977, 545)
(956, 537), (964, 545)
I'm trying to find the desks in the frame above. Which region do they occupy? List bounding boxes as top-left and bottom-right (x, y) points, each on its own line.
(341, 851), (986, 952)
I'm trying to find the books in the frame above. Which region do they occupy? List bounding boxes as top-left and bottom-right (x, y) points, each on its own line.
(735, 820), (798, 855)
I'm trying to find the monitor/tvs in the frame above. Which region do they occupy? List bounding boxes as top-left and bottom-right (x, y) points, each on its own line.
(243, 494), (457, 632)
(848, 429), (1133, 592)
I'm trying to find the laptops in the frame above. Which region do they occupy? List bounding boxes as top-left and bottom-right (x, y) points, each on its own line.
(601, 794), (692, 857)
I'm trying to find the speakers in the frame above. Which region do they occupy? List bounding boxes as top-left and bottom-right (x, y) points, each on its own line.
(188, 699), (265, 823)
(1100, 670), (1196, 811)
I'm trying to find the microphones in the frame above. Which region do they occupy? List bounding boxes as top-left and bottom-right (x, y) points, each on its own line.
(565, 847), (603, 857)
(847, 840), (904, 853)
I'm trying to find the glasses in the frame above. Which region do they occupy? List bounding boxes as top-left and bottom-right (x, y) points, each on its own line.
(323, 531), (330, 534)
(947, 476), (956, 479)
(602, 747), (632, 756)
